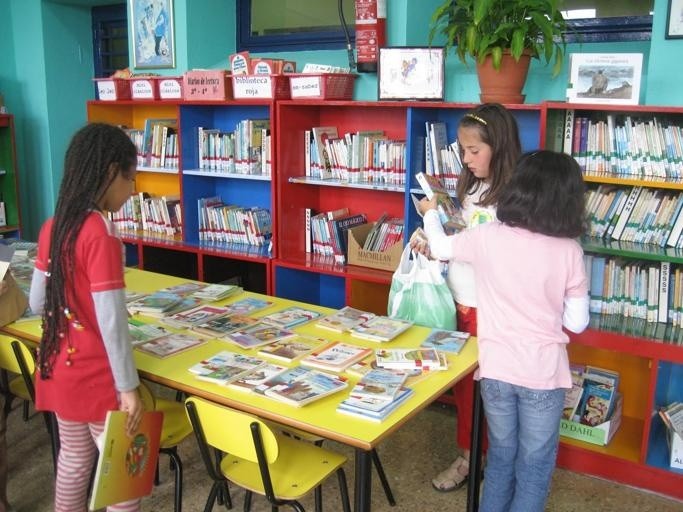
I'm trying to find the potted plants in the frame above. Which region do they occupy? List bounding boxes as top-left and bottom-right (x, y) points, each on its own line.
(426, 0), (569, 104)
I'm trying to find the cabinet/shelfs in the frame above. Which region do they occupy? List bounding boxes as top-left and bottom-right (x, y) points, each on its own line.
(270, 99), (683, 504)
(86, 98), (288, 294)
(0, 113), (23, 240)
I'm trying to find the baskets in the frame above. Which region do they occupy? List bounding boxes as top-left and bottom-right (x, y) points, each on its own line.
(225, 74), (288, 99)
(130, 77), (182, 101)
(283, 73), (359, 100)
(90, 78), (130, 100)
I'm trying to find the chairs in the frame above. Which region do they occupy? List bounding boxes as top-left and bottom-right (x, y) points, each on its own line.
(182, 394), (352, 512)
(110, 377), (195, 512)
(2, 334), (57, 478)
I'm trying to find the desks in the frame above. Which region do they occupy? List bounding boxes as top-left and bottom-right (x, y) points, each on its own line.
(0, 268), (484, 512)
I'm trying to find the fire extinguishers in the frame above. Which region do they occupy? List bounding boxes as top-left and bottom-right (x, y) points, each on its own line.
(338, 0), (385, 73)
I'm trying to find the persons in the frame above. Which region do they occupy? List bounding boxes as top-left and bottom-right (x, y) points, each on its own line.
(581, 69), (609, 96)
(28, 121), (144, 512)
(408, 102), (521, 490)
(418, 149), (591, 512)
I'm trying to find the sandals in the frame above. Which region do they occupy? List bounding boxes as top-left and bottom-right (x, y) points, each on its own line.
(431, 454), (470, 494)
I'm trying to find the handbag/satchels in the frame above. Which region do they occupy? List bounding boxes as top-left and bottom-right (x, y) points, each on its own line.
(386, 241), (457, 333)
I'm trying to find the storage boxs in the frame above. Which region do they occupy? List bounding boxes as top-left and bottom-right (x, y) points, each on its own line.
(183, 71), (232, 100)
(560, 392), (623, 447)
(347, 222), (404, 272)
(666, 428), (683, 470)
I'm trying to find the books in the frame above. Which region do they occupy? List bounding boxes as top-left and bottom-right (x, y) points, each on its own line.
(664, 404), (682, 439)
(0, 201), (7, 227)
(0, 234), (41, 324)
(302, 124), (464, 272)
(106, 117), (271, 247)
(561, 363), (619, 426)
(125, 281), (471, 422)
(658, 401), (678, 431)
(227, 47), (351, 97)
(88, 409), (163, 510)
(562, 110), (681, 331)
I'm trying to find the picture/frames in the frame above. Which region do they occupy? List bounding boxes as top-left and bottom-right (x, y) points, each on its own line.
(130, 0), (176, 69)
(567, 53), (644, 104)
(378, 46), (445, 101)
(665, 0), (683, 40)
